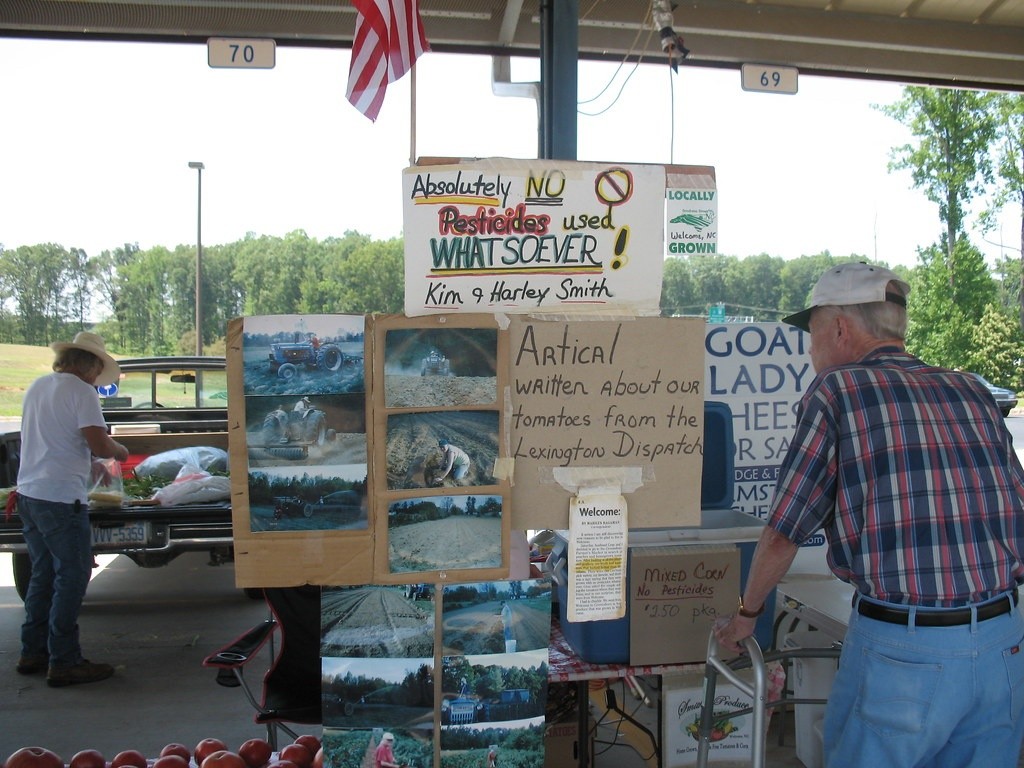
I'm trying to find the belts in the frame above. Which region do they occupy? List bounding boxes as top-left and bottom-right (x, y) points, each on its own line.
(851, 587), (1019, 627)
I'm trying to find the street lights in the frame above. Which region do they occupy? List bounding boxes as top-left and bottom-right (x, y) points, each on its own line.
(188, 162), (205, 409)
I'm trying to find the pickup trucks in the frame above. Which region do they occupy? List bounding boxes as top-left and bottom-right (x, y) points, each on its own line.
(1, 357), (268, 605)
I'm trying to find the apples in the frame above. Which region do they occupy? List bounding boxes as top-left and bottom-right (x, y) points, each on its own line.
(0, 734), (324, 768)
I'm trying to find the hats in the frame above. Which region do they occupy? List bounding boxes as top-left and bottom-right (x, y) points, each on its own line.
(301, 396), (310, 403)
(439, 439), (447, 445)
(383, 732), (393, 740)
(782, 261), (911, 333)
(465, 674), (468, 677)
(51, 331), (121, 386)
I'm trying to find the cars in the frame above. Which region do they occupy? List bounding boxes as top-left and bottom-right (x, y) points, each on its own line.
(972, 374), (1018, 418)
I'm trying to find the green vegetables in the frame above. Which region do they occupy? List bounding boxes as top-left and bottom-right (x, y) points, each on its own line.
(119, 465), (230, 498)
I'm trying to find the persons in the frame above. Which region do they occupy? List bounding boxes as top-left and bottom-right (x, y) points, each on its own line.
(291, 396), (311, 420)
(15, 330), (129, 688)
(310, 336), (320, 351)
(457, 674), (470, 701)
(319, 496), (325, 509)
(291, 495), (303, 504)
(374, 732), (401, 768)
(430, 350), (446, 362)
(433, 439), (471, 482)
(488, 745), (496, 768)
(712, 261), (1024, 768)
(273, 506), (283, 521)
(499, 601), (512, 643)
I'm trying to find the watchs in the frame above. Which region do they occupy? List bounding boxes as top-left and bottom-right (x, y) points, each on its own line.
(735, 593), (765, 618)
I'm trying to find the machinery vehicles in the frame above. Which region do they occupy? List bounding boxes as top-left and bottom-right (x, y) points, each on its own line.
(270, 332), (344, 380)
(274, 496), (312, 521)
(262, 408), (336, 448)
(442, 698), (492, 725)
(405, 583), (432, 602)
(322, 696), (354, 716)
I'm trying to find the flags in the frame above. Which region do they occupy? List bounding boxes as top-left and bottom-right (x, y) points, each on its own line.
(344, 0), (432, 124)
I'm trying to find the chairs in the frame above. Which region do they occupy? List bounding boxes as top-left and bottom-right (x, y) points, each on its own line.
(203, 582), (323, 754)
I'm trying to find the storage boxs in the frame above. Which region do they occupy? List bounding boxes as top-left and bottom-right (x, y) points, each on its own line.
(783, 631), (844, 768)
(544, 707), (598, 768)
(545, 506), (778, 665)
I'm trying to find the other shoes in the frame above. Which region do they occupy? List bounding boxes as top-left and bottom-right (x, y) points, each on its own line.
(47, 656), (114, 686)
(16, 654), (48, 674)
